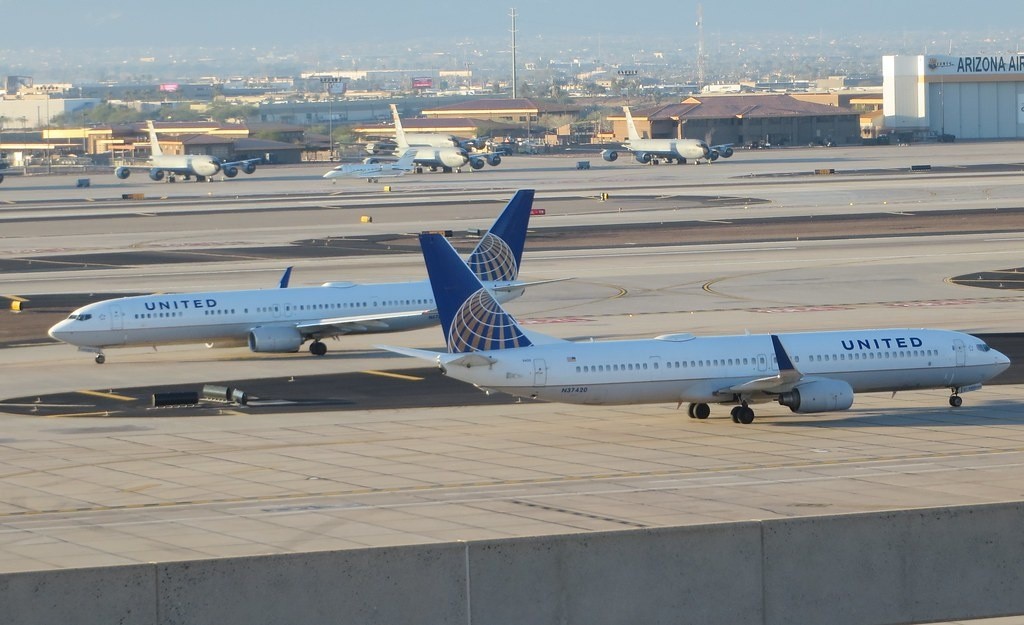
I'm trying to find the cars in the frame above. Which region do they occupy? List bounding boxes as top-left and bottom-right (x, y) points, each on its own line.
(495, 144), (512, 155)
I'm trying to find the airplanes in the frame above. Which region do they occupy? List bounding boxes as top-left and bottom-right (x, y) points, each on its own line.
(323, 104), (505, 184)
(601, 106), (735, 165)
(370, 233), (1013, 424)
(108, 120), (262, 182)
(45, 188), (579, 364)
